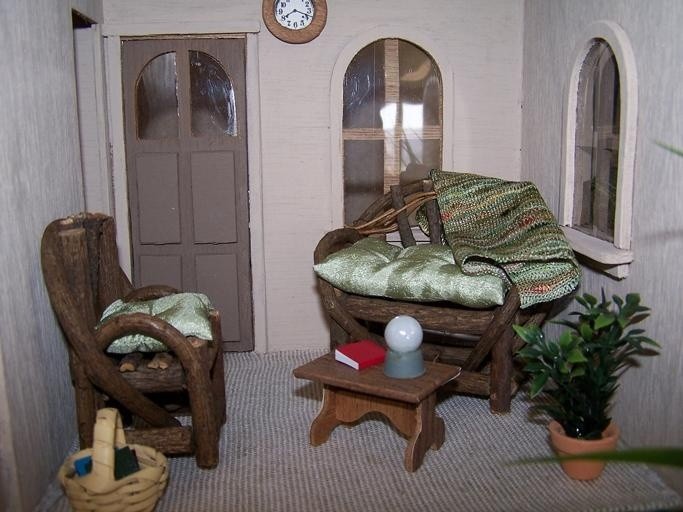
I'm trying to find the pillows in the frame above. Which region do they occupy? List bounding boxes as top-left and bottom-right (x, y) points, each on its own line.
(312, 236), (506, 310)
(98, 287), (214, 353)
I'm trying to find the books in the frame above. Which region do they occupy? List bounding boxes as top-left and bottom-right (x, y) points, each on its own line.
(334, 337), (386, 370)
(74, 446), (141, 481)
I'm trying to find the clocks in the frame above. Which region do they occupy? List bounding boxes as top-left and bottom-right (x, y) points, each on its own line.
(258, 1), (327, 45)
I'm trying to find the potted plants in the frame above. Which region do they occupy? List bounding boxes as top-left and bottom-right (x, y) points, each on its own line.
(511, 286), (662, 481)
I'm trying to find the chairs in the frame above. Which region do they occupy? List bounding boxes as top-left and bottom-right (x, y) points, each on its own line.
(313, 174), (578, 416)
(40, 212), (229, 469)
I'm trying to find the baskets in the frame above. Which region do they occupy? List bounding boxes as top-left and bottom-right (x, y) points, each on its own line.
(57, 406), (168, 512)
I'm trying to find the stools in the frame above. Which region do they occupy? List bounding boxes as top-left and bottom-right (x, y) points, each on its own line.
(288, 345), (463, 473)
